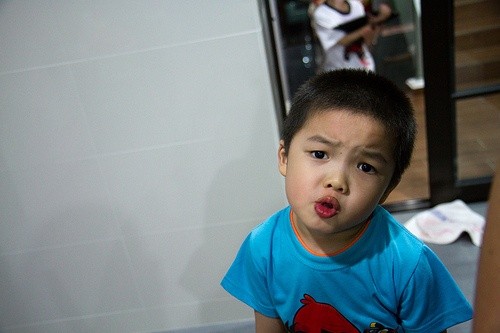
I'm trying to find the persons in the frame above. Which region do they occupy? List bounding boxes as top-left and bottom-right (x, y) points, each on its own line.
(312, 0), (394, 72)
(220, 69), (476, 333)
